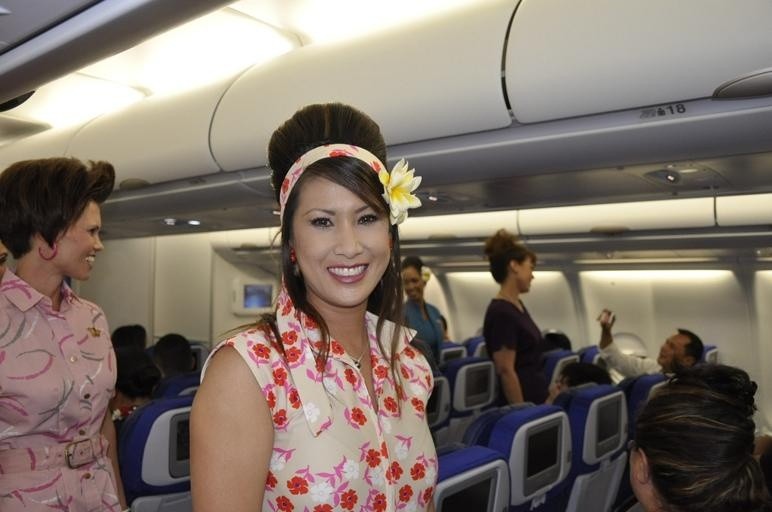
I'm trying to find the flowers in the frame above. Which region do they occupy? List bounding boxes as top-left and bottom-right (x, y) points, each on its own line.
(380, 155), (422, 225)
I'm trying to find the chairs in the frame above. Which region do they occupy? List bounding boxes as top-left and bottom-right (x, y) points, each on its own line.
(117, 396), (193, 511)
(158, 372), (199, 396)
(425, 334), (719, 511)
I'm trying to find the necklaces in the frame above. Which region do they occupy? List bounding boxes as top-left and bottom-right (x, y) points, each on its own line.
(346, 342), (370, 368)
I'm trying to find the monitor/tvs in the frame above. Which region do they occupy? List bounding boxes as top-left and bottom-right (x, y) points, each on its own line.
(424, 380), (442, 425)
(168, 411), (189, 479)
(436, 469), (498, 512)
(465, 365), (491, 408)
(230, 278), (278, 317)
(522, 414), (561, 497)
(593, 393), (623, 457)
(443, 350), (463, 365)
(190, 347), (201, 373)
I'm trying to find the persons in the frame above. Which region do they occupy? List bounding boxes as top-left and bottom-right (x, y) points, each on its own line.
(188, 103), (440, 511)
(110, 325), (192, 428)
(542, 361), (612, 406)
(0, 156), (129, 512)
(391, 254), (446, 371)
(545, 331), (572, 352)
(595, 308), (704, 391)
(480, 228), (550, 409)
(625, 362), (772, 511)
